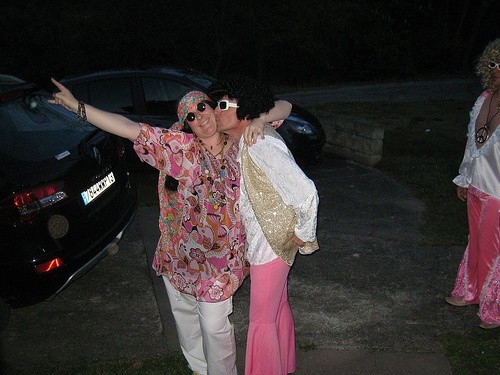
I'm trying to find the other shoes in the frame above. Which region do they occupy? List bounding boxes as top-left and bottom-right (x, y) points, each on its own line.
(478, 323), (500, 329)
(444, 296), (480, 307)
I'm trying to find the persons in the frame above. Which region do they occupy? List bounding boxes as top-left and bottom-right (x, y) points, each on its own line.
(204, 75), (319, 375)
(46, 78), (293, 375)
(441, 42), (500, 329)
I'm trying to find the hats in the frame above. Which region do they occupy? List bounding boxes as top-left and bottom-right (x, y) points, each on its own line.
(177, 91), (212, 124)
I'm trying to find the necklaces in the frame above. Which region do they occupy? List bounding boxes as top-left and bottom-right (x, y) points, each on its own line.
(203, 133), (226, 158)
(476, 89), (500, 144)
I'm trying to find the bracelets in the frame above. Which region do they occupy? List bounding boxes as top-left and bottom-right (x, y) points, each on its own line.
(77, 101), (87, 122)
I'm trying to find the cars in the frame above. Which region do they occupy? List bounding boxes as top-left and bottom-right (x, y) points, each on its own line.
(40, 66), (327, 186)
(0, 75), (138, 333)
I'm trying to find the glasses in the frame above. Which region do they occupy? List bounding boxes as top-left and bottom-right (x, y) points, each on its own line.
(186, 102), (206, 122)
(488, 61), (500, 71)
(217, 99), (240, 111)
